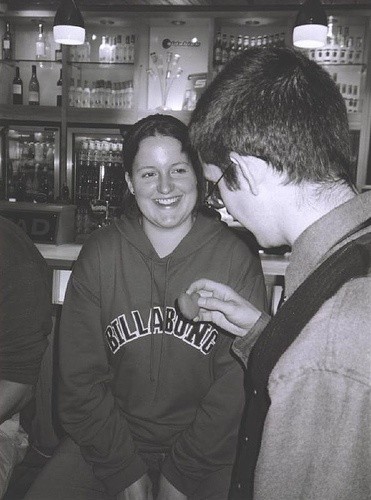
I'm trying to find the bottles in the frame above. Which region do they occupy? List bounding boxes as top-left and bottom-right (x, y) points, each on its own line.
(67, 77), (134, 110)
(12, 67), (23, 105)
(73, 137), (126, 207)
(35, 24), (45, 59)
(55, 43), (64, 60)
(57, 68), (63, 107)
(3, 22), (12, 61)
(6, 129), (57, 202)
(69, 34), (137, 63)
(29, 65), (40, 105)
(213, 31), (287, 67)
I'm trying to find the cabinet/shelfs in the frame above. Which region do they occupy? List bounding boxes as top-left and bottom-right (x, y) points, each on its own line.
(1, 0), (371, 253)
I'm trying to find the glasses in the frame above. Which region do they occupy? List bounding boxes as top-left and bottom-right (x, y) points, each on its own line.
(203, 162), (234, 209)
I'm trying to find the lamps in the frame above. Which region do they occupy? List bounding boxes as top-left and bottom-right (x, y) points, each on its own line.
(291, 0), (329, 49)
(52, 0), (87, 47)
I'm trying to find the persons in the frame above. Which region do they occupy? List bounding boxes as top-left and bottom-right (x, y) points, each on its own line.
(25, 113), (268, 500)
(186, 39), (371, 500)
(0, 215), (53, 500)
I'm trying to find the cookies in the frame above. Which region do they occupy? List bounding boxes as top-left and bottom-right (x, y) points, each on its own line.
(178, 292), (202, 319)
